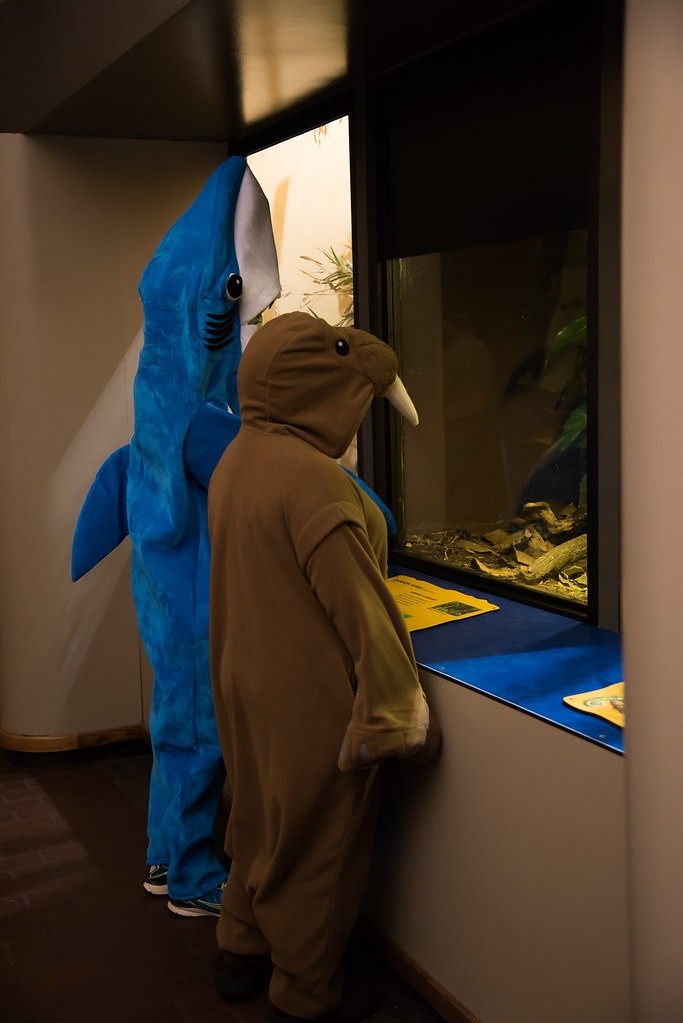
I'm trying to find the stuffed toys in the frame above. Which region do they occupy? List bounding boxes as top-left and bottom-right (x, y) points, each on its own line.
(215, 310), (442, 1023)
(73, 157), (282, 918)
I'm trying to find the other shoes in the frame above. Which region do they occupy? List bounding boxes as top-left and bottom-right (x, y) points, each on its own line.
(208, 947), (269, 1002)
(270, 978), (381, 1023)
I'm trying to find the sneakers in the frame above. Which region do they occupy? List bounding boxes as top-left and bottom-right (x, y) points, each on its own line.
(142, 863), (171, 897)
(167, 879), (229, 919)
